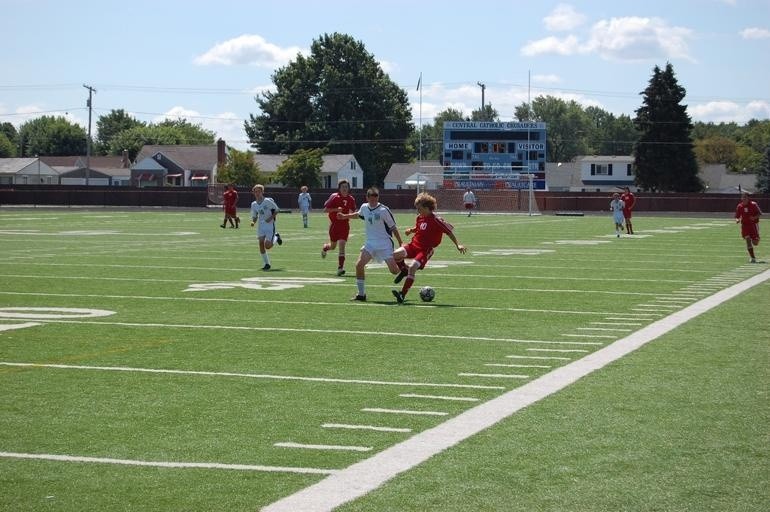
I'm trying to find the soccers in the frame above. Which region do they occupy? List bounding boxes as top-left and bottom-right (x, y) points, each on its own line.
(420, 286), (435, 301)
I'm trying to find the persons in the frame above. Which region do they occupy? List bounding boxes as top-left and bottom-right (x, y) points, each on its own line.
(392, 191), (467, 304)
(220, 183), (239, 228)
(250, 183), (282, 270)
(463, 188), (475, 217)
(734, 191), (762, 263)
(297, 185), (312, 228)
(336, 186), (404, 301)
(222, 186), (235, 228)
(321, 179), (357, 277)
(610, 192), (625, 237)
(619, 186), (636, 234)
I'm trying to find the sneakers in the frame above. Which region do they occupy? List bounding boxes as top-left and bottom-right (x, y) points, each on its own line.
(351, 293), (367, 301)
(220, 224), (225, 228)
(262, 264), (270, 270)
(395, 270), (407, 283)
(393, 290), (404, 302)
(275, 233), (282, 245)
(322, 250), (327, 258)
(338, 269), (345, 276)
